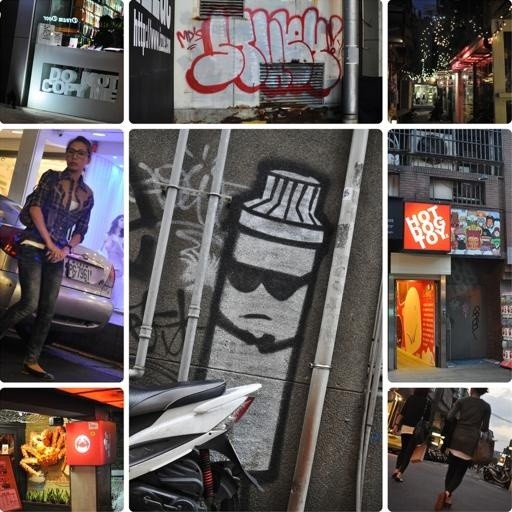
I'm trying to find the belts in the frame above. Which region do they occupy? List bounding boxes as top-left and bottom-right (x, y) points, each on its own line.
(20, 239), (45, 250)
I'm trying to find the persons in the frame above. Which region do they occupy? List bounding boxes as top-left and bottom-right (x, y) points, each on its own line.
(111, 14), (122, 47)
(391, 388), (433, 482)
(98, 214), (124, 310)
(434, 389), (491, 512)
(1, 136), (95, 378)
(93, 13), (114, 47)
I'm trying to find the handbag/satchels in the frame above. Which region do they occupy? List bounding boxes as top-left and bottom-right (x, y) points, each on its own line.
(18, 188), (35, 227)
(396, 414), (406, 426)
(472, 429), (495, 465)
(410, 420), (428, 463)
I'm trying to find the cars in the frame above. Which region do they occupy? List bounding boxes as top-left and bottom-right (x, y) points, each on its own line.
(0, 194), (115, 345)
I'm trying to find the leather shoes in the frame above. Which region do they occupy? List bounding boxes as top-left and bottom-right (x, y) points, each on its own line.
(436, 491), (452, 511)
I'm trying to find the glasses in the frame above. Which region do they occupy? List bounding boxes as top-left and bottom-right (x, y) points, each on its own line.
(67, 148), (90, 156)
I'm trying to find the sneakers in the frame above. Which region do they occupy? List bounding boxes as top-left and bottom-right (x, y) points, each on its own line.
(22, 361), (54, 380)
(392, 469), (404, 482)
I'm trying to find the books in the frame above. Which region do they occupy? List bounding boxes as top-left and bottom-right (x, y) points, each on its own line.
(63, 0), (123, 44)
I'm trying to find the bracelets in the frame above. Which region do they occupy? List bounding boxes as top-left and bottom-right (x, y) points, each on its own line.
(394, 423), (399, 426)
(65, 243), (72, 255)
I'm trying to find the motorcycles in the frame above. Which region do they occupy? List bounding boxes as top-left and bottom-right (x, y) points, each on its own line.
(484, 466), (511, 490)
(129, 379), (266, 512)
